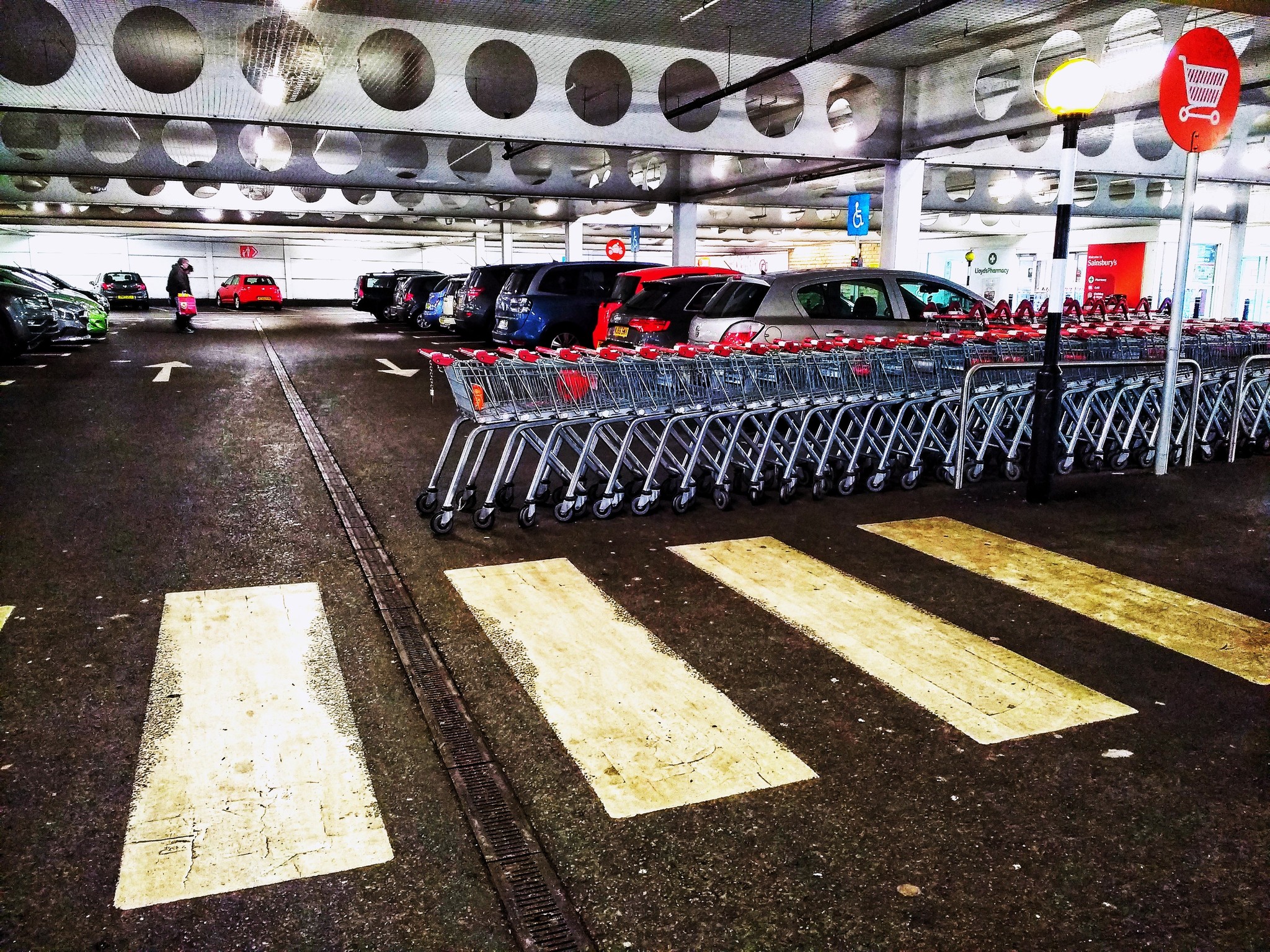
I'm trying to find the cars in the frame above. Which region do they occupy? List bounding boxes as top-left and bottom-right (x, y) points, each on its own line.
(89, 270), (150, 310)
(0, 265), (112, 363)
(423, 272), (470, 333)
(215, 274), (283, 310)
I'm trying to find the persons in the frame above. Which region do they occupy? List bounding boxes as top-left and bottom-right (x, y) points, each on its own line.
(166, 257), (197, 333)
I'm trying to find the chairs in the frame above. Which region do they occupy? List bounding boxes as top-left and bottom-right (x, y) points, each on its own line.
(124, 275), (131, 281)
(852, 296), (877, 320)
(107, 276), (113, 280)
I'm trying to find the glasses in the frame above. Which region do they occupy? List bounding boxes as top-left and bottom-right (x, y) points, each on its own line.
(186, 263), (188, 265)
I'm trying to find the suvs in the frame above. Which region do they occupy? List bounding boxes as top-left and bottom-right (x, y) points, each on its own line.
(451, 261), (1024, 355)
(351, 269), (449, 332)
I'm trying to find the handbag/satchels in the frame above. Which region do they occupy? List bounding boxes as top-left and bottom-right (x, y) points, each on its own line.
(175, 291), (198, 316)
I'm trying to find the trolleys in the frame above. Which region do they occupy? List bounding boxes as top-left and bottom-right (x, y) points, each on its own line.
(413, 315), (1270, 536)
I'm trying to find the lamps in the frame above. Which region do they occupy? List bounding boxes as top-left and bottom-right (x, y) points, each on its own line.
(965, 252), (975, 262)
(1044, 59), (1106, 122)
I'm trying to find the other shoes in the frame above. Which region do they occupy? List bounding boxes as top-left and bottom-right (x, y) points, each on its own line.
(181, 328), (195, 332)
